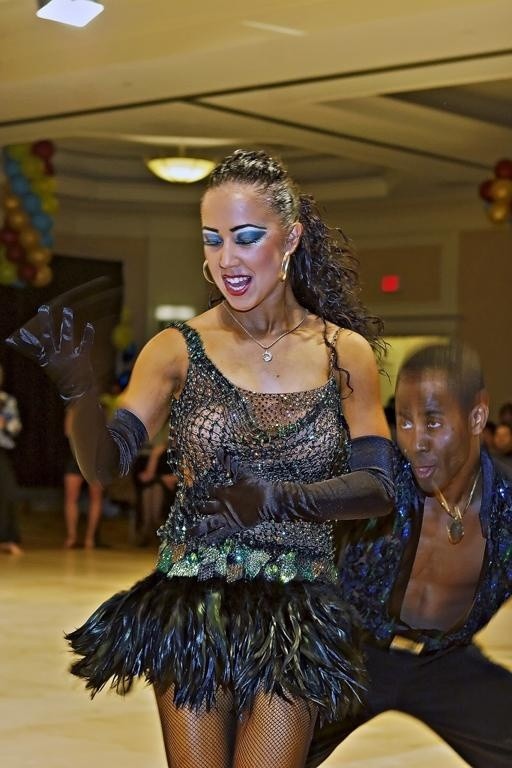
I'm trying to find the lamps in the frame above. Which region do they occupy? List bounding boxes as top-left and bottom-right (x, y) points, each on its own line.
(147, 145), (219, 185)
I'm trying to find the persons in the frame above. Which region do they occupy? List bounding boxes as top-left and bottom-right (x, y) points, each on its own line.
(6, 150), (398, 768)
(481, 404), (512, 471)
(307, 342), (511, 768)
(61, 342), (178, 549)
(0, 366), (24, 555)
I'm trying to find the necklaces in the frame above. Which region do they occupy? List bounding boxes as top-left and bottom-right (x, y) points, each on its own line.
(436, 468), (483, 545)
(222, 301), (309, 363)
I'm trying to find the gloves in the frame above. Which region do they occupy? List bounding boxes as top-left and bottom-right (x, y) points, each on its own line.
(4, 304), (149, 492)
(186, 436), (397, 548)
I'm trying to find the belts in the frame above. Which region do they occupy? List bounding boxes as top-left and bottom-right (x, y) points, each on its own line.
(352, 628), (452, 658)
